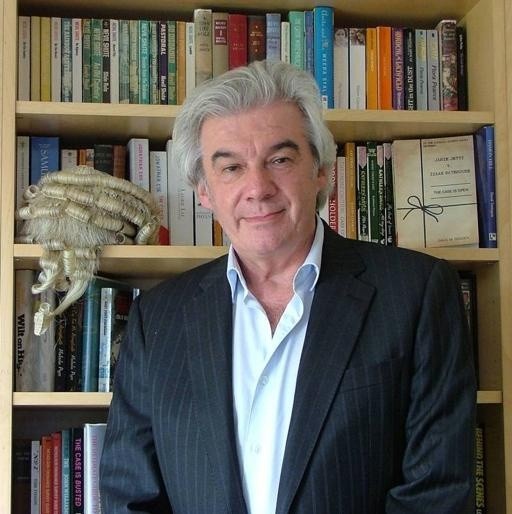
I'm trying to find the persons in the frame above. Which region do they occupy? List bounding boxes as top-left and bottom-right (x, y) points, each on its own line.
(99, 58), (476, 514)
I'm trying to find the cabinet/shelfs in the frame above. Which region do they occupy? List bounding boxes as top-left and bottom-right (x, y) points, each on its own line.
(0, 1), (512, 513)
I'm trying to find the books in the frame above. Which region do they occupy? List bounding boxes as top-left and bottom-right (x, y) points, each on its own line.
(11, 423), (107, 514)
(458, 268), (473, 328)
(15, 269), (148, 392)
(316, 123), (497, 247)
(16, 136), (232, 247)
(473, 424), (486, 514)
